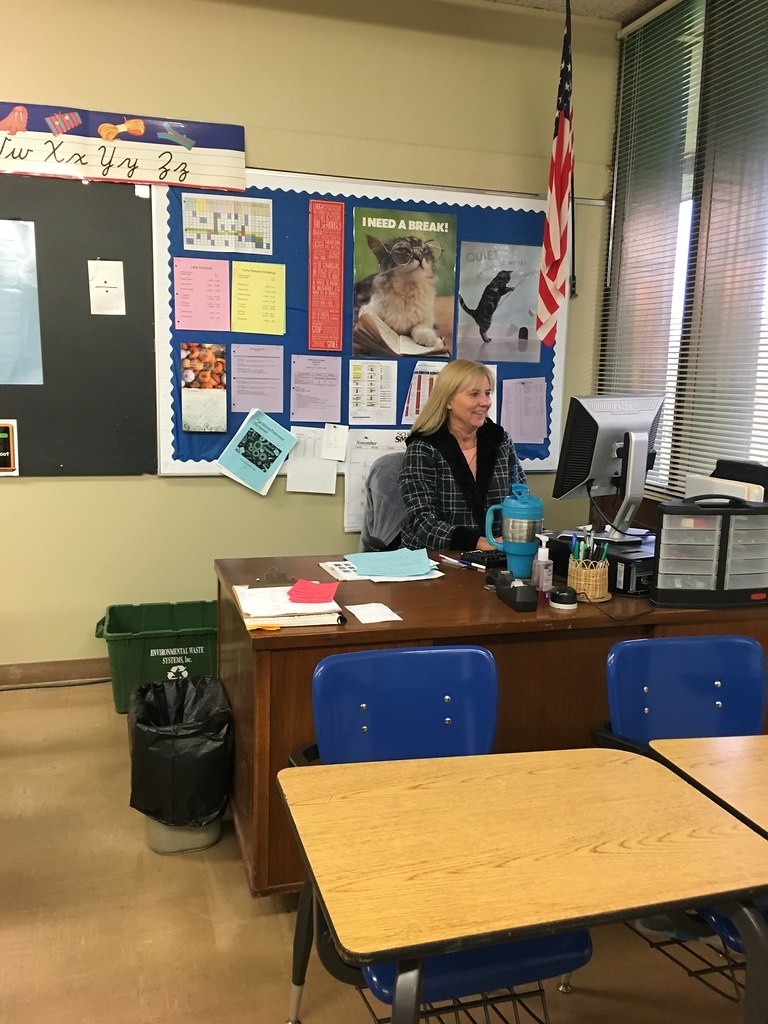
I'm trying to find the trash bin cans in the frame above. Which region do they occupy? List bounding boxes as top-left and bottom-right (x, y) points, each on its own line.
(129, 678), (232, 854)
(97, 600), (222, 715)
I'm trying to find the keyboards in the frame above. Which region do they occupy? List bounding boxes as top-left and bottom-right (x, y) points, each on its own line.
(459, 550), (506, 569)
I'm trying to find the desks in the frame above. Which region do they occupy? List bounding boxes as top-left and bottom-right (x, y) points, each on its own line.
(275, 749), (768, 1024)
(649, 735), (768, 839)
(212, 556), (768, 900)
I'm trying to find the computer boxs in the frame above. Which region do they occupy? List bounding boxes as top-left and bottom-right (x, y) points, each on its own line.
(542, 533), (658, 597)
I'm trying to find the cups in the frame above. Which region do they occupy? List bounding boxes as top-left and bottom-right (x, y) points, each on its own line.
(486, 485), (544, 579)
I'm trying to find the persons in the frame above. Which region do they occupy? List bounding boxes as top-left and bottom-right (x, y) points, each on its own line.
(394, 359), (554, 552)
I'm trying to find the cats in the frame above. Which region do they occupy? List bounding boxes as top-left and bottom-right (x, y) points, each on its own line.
(353, 232), (440, 355)
(458, 270), (515, 343)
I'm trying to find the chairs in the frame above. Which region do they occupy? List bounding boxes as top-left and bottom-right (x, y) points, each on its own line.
(291, 647), (549, 1024)
(600, 637), (768, 1001)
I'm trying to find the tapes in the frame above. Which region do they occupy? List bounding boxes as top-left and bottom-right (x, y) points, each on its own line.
(511, 579), (524, 589)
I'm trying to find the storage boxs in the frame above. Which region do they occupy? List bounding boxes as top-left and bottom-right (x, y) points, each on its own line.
(96, 599), (216, 714)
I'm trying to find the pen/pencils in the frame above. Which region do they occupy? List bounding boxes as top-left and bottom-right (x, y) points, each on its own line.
(439, 554), (486, 571)
(571, 527), (609, 568)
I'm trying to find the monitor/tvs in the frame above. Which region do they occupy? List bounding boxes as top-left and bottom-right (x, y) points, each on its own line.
(552, 392), (666, 544)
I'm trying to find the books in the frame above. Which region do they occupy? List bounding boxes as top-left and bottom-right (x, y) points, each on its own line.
(230, 579), (347, 632)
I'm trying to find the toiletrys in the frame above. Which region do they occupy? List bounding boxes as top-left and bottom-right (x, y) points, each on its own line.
(531, 533), (555, 591)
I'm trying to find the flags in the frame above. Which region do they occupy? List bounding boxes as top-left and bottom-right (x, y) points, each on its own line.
(536, 10), (574, 351)
